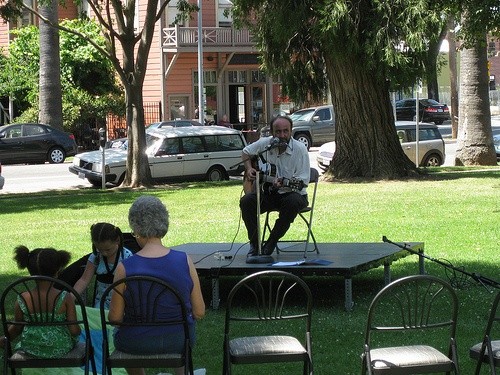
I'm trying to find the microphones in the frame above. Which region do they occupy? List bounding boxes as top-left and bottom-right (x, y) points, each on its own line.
(268, 137), (280, 151)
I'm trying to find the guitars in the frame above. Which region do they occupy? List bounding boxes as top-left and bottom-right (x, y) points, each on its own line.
(243, 154), (306, 195)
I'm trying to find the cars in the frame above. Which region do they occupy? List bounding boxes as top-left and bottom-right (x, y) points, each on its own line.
(396, 98), (450, 125)
(1, 121), (77, 165)
(316, 120), (446, 172)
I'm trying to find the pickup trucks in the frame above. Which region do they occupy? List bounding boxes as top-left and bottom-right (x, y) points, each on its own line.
(69, 125), (251, 190)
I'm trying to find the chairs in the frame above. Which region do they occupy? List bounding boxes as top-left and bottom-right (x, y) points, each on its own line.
(470, 289), (500, 375)
(224, 269), (314, 375)
(100, 275), (194, 375)
(0, 275), (97, 375)
(359, 275), (459, 375)
(262, 168), (321, 258)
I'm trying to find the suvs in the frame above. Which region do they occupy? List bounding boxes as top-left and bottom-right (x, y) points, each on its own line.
(110, 120), (203, 147)
(260, 106), (335, 152)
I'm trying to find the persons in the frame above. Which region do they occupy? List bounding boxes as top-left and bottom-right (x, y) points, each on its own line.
(108, 197), (206, 375)
(239, 116), (310, 255)
(0, 245), (82, 358)
(70, 222), (133, 310)
(218, 114), (232, 128)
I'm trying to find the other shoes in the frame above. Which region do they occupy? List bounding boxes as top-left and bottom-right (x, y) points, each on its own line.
(247, 242), (259, 257)
(262, 238), (277, 255)
